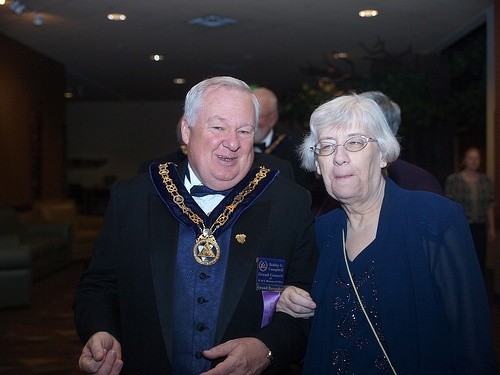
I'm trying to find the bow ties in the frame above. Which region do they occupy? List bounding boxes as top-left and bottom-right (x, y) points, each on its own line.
(190, 185), (234, 197)
(252, 141), (267, 153)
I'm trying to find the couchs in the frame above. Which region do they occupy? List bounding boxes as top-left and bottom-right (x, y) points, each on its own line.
(17, 201), (106, 260)
(1, 197), (74, 308)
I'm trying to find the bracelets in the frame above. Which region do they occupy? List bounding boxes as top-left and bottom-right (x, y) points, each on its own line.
(267, 348), (274, 361)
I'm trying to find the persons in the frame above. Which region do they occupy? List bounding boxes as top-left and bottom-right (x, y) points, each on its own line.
(251, 88), (318, 196)
(72, 76), (312, 374)
(275, 94), (492, 375)
(444, 148), (494, 267)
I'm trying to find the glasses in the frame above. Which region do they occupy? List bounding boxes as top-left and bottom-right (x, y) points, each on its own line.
(310, 135), (377, 155)
